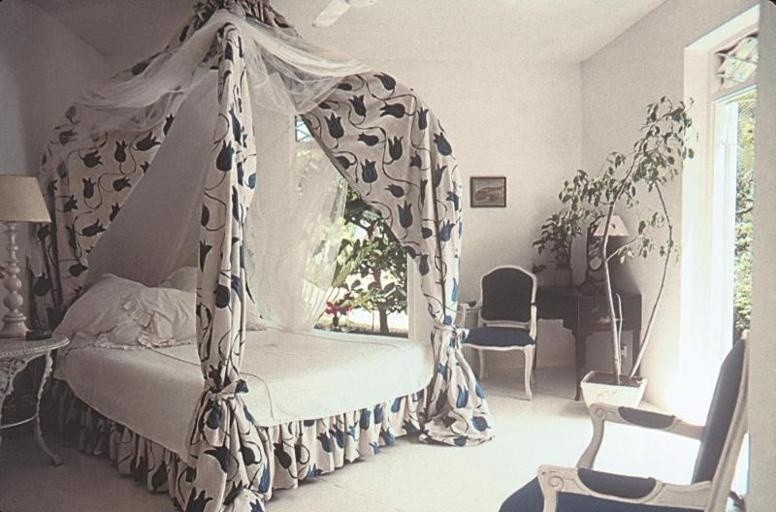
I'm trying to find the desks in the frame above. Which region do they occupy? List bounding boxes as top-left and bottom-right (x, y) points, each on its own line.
(535, 284), (642, 402)
(0, 334), (70, 468)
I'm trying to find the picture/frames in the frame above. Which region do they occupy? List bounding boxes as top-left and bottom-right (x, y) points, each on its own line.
(467, 174), (508, 209)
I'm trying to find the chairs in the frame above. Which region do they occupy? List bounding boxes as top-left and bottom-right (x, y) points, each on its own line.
(498, 325), (750, 512)
(458, 262), (539, 400)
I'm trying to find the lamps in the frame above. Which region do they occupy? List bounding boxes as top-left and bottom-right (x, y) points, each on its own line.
(0, 171), (54, 340)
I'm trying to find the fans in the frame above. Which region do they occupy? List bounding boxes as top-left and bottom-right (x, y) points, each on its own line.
(313, 0), (378, 28)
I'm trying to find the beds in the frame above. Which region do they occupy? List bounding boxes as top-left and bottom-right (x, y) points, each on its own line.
(26, 1), (498, 511)
